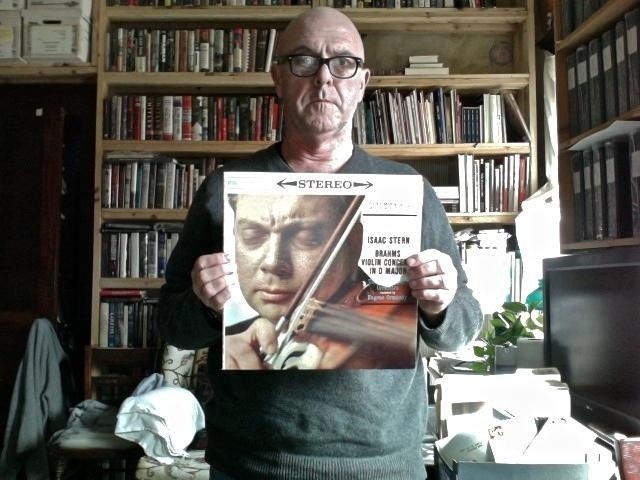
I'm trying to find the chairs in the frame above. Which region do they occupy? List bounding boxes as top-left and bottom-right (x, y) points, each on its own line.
(47, 345), (158, 480)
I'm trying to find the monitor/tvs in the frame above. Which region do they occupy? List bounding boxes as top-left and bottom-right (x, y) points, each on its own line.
(543, 247), (640, 437)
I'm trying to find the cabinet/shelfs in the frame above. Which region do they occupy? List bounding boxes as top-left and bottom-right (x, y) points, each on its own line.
(0, 1), (98, 84)
(318, 0), (538, 326)
(554, 0), (640, 253)
(90, 0), (318, 406)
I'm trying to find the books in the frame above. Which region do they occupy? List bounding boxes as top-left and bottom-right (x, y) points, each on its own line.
(102, 0), (286, 72)
(101, 152), (226, 208)
(221, 171), (424, 370)
(102, 221), (184, 348)
(404, 54), (449, 74)
(350, 87), (529, 143)
(555, 0), (639, 242)
(288, 1), (485, 8)
(432, 154), (530, 313)
(103, 94), (284, 142)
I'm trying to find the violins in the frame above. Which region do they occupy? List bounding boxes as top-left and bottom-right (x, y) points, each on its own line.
(259, 282), (417, 370)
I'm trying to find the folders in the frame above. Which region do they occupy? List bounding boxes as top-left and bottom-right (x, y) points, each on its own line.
(563, 9), (640, 134)
(558, 133), (640, 242)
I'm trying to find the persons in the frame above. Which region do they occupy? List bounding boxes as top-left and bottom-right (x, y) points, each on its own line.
(226, 194), (416, 370)
(156, 7), (482, 479)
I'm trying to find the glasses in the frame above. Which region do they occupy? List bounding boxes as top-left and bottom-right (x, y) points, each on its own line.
(278, 55), (365, 79)
